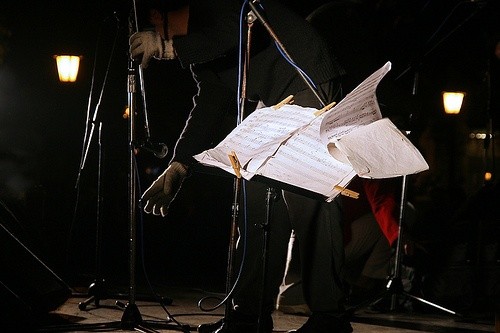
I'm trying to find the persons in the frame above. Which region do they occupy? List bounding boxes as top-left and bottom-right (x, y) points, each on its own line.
(345, 196), (400, 308)
(124, 1), (353, 332)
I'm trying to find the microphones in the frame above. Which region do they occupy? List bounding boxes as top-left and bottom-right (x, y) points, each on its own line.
(100, 7), (121, 29)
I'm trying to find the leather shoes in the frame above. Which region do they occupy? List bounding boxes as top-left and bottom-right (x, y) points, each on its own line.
(198, 305), (276, 332)
(295, 314), (355, 333)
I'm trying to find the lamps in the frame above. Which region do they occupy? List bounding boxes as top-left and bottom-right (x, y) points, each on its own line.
(52, 54), (83, 85)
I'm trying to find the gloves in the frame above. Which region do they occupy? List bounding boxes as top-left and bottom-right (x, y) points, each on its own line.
(129, 30), (176, 69)
(139, 155), (192, 218)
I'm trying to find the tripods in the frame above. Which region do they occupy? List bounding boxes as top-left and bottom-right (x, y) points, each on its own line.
(341, 4), (483, 324)
(203, 1), (331, 333)
(35, 0), (195, 333)
(59, 26), (173, 312)
